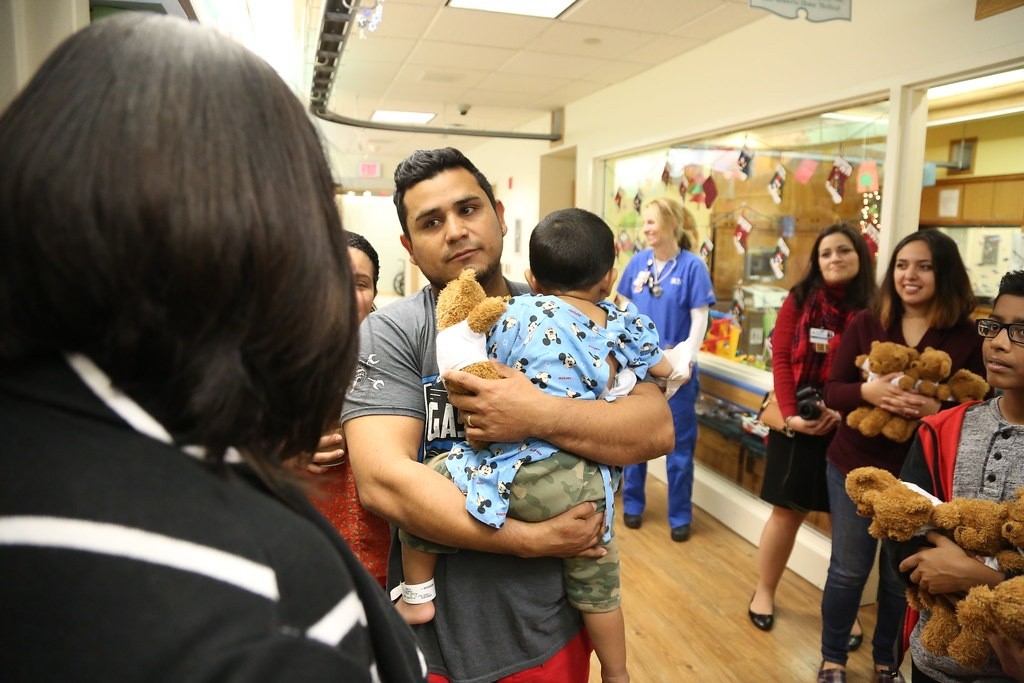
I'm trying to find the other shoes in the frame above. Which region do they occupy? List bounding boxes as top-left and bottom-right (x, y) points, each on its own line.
(874, 664), (906, 683)
(818, 660), (847, 683)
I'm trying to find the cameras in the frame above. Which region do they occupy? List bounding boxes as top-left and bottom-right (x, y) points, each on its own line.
(797, 386), (824, 420)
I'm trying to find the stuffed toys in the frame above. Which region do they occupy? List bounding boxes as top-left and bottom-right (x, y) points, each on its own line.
(435, 269), (511, 452)
(843, 466), (1024, 683)
(847, 338), (990, 441)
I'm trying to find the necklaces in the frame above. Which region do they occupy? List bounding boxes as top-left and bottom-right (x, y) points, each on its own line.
(651, 246), (679, 298)
(554, 293), (593, 303)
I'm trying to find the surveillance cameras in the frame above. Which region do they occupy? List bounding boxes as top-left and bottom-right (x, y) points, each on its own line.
(456, 105), (470, 115)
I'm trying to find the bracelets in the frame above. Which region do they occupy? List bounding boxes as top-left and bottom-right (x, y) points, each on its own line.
(785, 417), (795, 438)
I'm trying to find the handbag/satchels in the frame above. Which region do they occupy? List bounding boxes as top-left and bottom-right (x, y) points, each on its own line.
(760, 393), (794, 437)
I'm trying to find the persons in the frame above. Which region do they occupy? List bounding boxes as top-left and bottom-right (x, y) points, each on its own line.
(0, 12), (430, 683)
(885, 271), (1024, 683)
(748, 224), (876, 653)
(615, 198), (715, 542)
(813, 227), (986, 683)
(274, 147), (674, 683)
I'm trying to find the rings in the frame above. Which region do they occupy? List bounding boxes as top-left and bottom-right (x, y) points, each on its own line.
(467, 414), (475, 427)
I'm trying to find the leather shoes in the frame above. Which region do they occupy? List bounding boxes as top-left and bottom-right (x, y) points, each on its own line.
(748, 589), (773, 632)
(847, 617), (863, 650)
(672, 524), (689, 542)
(624, 510), (642, 528)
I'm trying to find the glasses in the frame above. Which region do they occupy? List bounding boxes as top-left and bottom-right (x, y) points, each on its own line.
(975, 317), (1024, 343)
